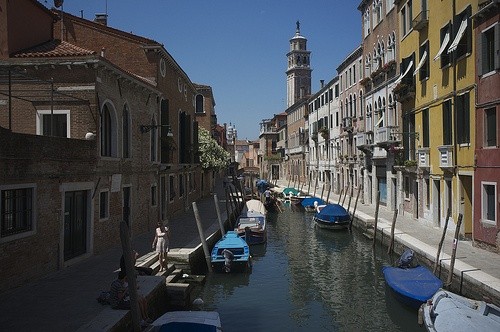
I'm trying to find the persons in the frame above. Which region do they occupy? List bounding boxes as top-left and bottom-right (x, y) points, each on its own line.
(110, 271), (130, 310)
(152, 221), (171, 271)
(263, 187), (272, 206)
(120, 248), (138, 282)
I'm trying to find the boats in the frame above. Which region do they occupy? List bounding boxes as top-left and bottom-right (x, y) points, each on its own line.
(142, 310), (223, 332)
(418, 288), (500, 332)
(312, 202), (350, 230)
(233, 178), (327, 246)
(382, 246), (444, 303)
(209, 230), (251, 267)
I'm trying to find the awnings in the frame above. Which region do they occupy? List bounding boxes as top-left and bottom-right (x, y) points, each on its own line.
(393, 56), (414, 84)
(447, 14), (467, 54)
(413, 44), (427, 76)
(434, 30), (449, 62)
